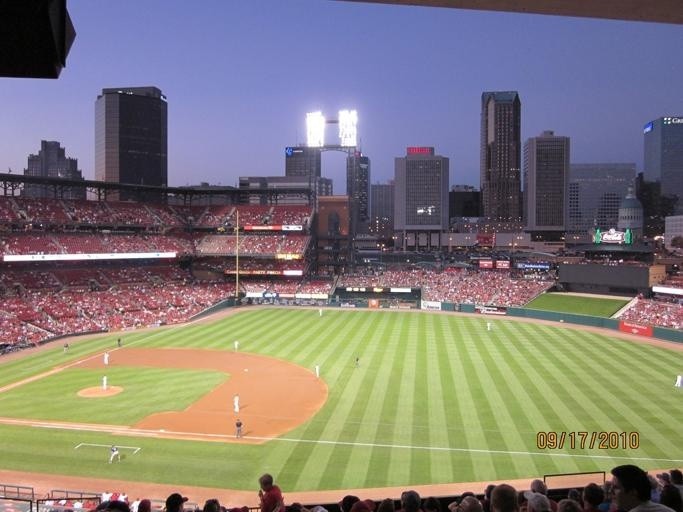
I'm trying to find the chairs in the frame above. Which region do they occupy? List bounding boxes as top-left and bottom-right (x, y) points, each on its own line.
(199, 199), (318, 278)
(340, 269), (556, 307)
(615, 273), (683, 332)
(242, 267), (341, 307)
(2, 193), (198, 257)
(1, 265), (230, 349)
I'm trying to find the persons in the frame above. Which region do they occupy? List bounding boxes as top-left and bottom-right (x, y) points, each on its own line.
(101, 373), (107, 389)
(354, 357), (359, 366)
(314, 364), (319, 378)
(108, 444), (119, 463)
(232, 393), (239, 414)
(674, 373), (681, 388)
(49, 464), (683, 511)
(103, 351), (109, 367)
(620, 291), (682, 330)
(0, 195), (332, 354)
(337, 265), (553, 309)
(234, 418), (242, 438)
(486, 319), (492, 331)
